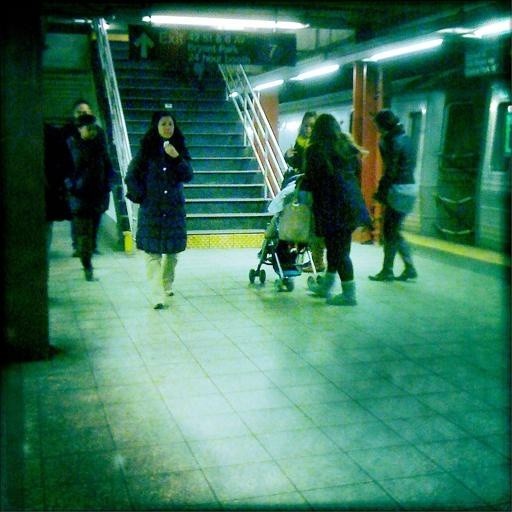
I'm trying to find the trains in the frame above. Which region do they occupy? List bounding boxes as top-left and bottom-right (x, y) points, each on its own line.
(242, 74), (511, 256)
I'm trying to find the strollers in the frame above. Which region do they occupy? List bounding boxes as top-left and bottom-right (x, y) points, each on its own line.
(248, 174), (326, 293)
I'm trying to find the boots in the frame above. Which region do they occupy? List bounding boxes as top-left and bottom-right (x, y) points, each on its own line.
(310, 272), (336, 298)
(326, 278), (359, 307)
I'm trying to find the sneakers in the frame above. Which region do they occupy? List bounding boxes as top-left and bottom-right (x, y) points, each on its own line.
(153, 287), (175, 310)
(393, 267), (418, 282)
(367, 267), (394, 281)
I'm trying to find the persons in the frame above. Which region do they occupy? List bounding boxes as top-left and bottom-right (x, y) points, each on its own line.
(129, 109), (195, 311)
(51, 98), (109, 261)
(294, 114), (372, 309)
(56, 113), (115, 285)
(282, 109), (326, 275)
(367, 108), (420, 283)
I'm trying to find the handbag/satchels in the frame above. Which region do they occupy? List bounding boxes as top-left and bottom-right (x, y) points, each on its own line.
(384, 182), (421, 216)
(125, 162), (149, 204)
(278, 201), (317, 243)
(280, 161), (297, 190)
